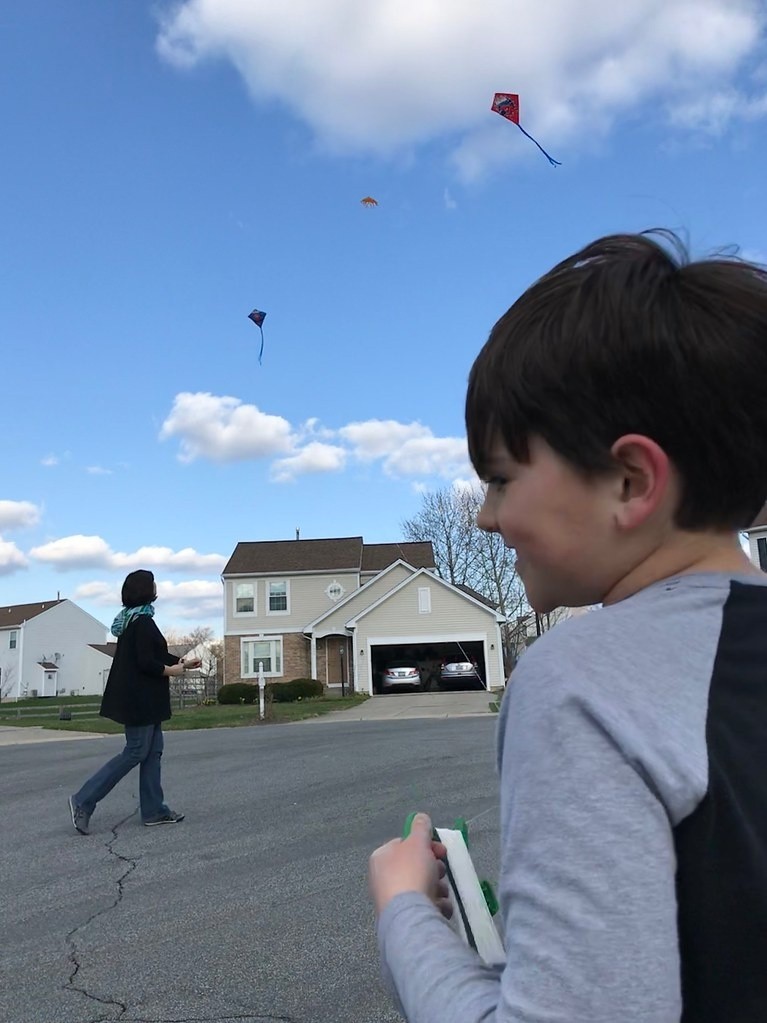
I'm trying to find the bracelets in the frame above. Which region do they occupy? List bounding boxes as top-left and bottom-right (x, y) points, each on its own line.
(181, 659), (184, 663)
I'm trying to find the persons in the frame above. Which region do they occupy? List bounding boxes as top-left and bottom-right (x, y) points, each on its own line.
(69, 570), (202, 834)
(370, 231), (766, 1023)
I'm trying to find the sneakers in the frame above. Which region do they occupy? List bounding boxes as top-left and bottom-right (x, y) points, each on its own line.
(68, 795), (92, 835)
(144, 804), (186, 826)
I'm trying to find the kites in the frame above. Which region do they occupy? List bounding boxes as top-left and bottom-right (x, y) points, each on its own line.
(491, 92), (562, 168)
(360, 196), (379, 208)
(248, 308), (267, 366)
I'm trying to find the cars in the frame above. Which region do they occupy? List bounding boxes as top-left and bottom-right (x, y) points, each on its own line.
(383, 661), (430, 692)
(441, 652), (480, 690)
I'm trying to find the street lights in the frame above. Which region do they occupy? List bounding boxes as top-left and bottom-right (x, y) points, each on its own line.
(338, 644), (344, 697)
